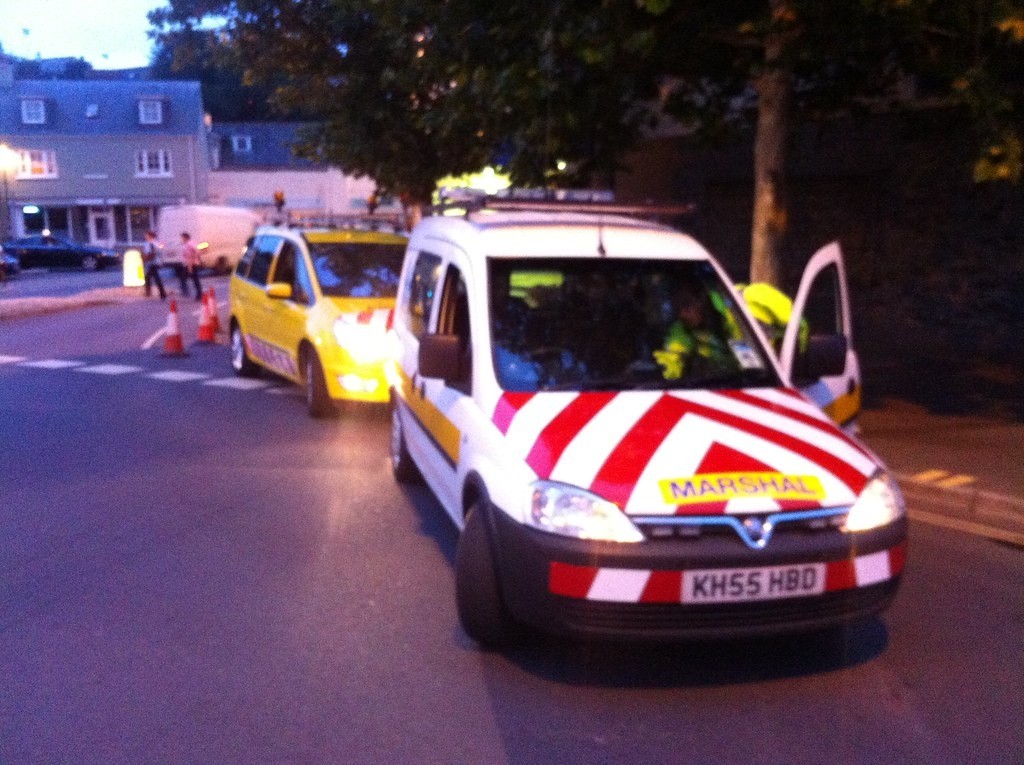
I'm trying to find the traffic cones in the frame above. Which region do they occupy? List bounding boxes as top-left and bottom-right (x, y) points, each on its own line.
(157, 299), (191, 359)
(196, 290), (220, 347)
(205, 285), (223, 331)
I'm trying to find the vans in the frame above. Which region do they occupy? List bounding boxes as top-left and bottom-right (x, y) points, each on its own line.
(383, 186), (909, 654)
(155, 202), (267, 274)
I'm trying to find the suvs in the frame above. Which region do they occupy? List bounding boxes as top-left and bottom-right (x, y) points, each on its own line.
(225, 191), (443, 417)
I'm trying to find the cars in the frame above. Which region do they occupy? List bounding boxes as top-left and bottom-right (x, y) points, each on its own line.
(1, 228), (120, 271)
(0, 244), (23, 280)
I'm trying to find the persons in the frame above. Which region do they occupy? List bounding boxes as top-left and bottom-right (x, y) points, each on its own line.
(179, 232), (203, 301)
(263, 190), (300, 229)
(142, 230), (167, 300)
(655, 281), (810, 380)
